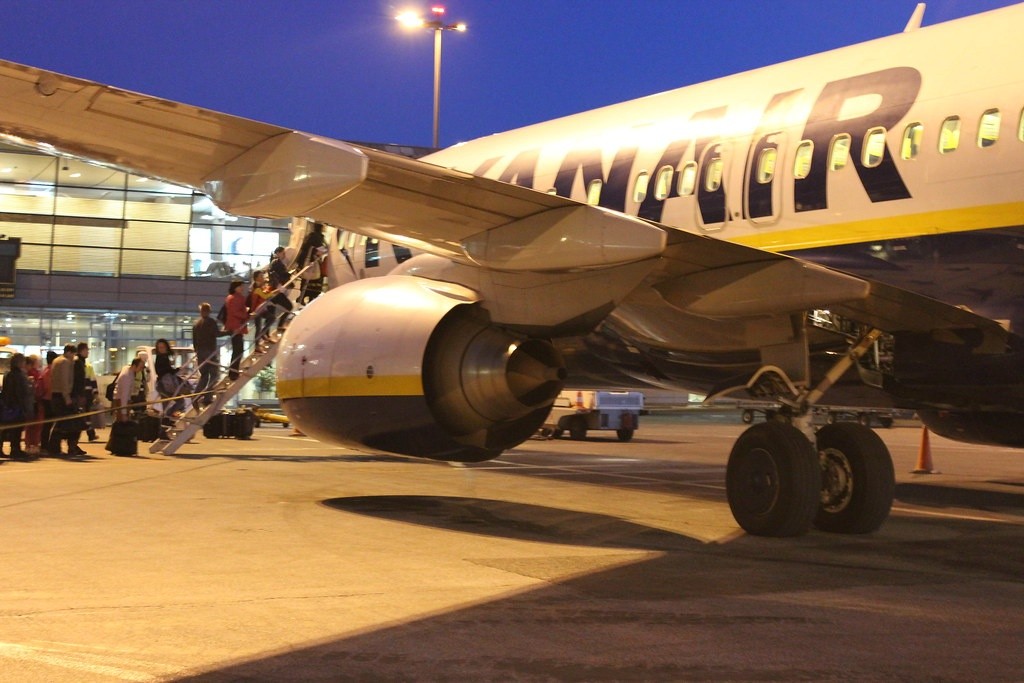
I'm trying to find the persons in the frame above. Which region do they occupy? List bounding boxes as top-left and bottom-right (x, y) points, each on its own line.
(259, 246), (294, 337)
(247, 269), (280, 354)
(219, 280), (249, 379)
(0, 341), (88, 462)
(109, 356), (144, 423)
(84, 362), (98, 441)
(192, 302), (232, 410)
(294, 222), (326, 307)
(129, 350), (152, 412)
(153, 337), (186, 441)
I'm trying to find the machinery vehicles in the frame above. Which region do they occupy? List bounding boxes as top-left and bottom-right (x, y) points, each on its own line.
(539, 388), (650, 444)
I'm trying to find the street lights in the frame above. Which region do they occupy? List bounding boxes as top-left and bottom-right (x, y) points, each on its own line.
(394, 2), (468, 150)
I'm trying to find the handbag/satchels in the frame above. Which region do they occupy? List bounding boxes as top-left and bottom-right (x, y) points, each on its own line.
(88, 401), (106, 428)
(161, 373), (193, 394)
(301, 246), (320, 280)
(105, 372), (122, 401)
(217, 304), (227, 325)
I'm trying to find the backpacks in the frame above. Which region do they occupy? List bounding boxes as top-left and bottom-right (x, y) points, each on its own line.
(244, 286), (263, 315)
(32, 368), (51, 396)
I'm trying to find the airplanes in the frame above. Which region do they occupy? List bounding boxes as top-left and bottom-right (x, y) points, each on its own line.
(0, 2), (1023, 537)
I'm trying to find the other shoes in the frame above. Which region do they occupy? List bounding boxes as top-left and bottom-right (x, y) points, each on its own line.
(229, 374), (239, 380)
(296, 296), (306, 306)
(277, 327), (286, 332)
(25, 446), (40, 456)
(50, 451), (67, 457)
(202, 400), (213, 405)
(255, 346), (268, 354)
(162, 420), (176, 427)
(160, 435), (173, 441)
(10, 451), (26, 457)
(0, 450), (10, 458)
(39, 449), (51, 457)
(67, 447), (86, 456)
(191, 397), (199, 411)
(265, 338), (278, 344)
(89, 436), (99, 441)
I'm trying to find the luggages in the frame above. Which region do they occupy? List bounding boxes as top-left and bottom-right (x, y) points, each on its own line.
(132, 409), (172, 442)
(105, 421), (139, 456)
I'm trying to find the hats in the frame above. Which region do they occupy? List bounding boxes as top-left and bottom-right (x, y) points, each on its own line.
(199, 303), (212, 312)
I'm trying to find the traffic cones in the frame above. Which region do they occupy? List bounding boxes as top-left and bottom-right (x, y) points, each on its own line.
(575, 391), (587, 410)
(909, 423), (942, 476)
